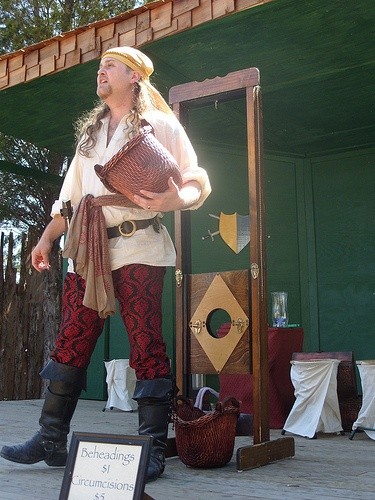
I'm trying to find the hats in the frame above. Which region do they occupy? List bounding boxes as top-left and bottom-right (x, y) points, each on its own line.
(100, 46), (155, 81)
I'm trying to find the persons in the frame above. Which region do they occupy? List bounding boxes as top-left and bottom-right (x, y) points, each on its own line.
(0, 46), (213, 484)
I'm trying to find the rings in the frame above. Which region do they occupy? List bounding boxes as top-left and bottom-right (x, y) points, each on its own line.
(148, 206), (150, 209)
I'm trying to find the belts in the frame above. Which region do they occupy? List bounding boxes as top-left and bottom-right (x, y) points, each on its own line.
(106, 218), (155, 239)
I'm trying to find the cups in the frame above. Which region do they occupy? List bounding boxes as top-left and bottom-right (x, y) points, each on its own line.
(271, 292), (288, 328)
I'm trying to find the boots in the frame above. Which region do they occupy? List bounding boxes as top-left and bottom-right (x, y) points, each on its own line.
(0, 358), (88, 467)
(132, 376), (179, 483)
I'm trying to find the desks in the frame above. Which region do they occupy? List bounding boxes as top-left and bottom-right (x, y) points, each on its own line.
(216, 321), (304, 429)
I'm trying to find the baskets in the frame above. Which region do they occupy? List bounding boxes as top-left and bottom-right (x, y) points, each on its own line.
(167, 394), (241, 471)
(94, 119), (183, 204)
